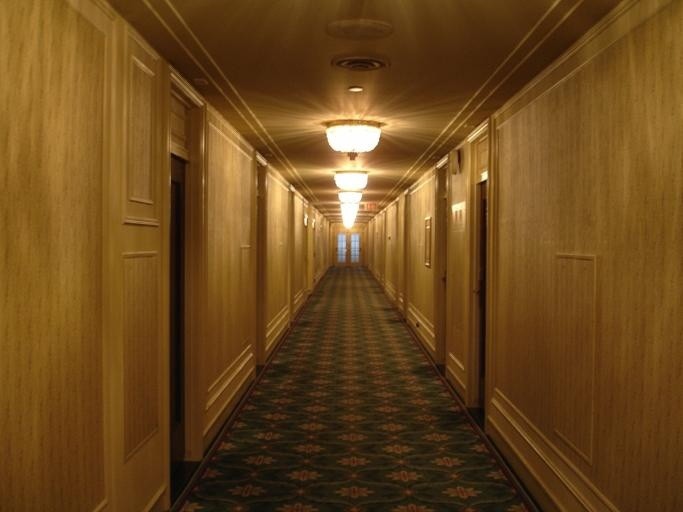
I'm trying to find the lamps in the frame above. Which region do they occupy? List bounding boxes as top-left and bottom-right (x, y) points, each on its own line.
(324, 119), (382, 190)
